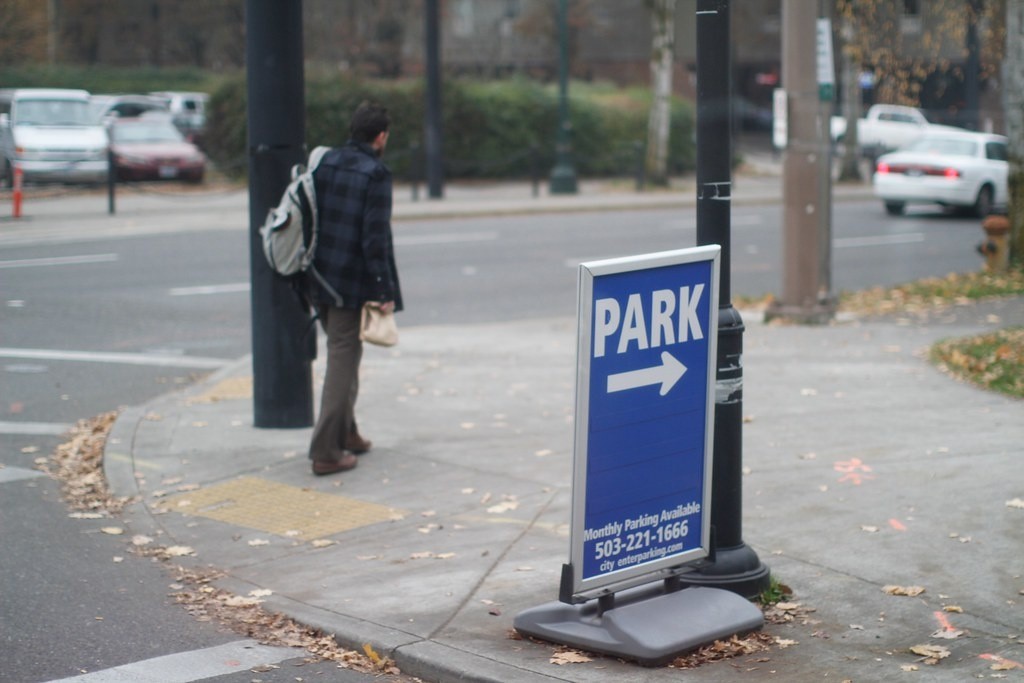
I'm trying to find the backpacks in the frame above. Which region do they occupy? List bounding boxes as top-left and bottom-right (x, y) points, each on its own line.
(259, 146), (331, 276)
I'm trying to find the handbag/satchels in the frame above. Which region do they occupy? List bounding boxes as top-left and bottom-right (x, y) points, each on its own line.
(360, 300), (398, 348)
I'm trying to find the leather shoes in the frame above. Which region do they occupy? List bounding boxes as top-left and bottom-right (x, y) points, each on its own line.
(312, 455), (356, 475)
(340, 433), (371, 455)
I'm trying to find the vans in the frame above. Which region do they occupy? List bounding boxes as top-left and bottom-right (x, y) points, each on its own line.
(0, 88), (109, 186)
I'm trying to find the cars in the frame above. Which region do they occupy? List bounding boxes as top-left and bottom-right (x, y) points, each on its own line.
(874, 129), (1010, 220)
(109, 117), (206, 185)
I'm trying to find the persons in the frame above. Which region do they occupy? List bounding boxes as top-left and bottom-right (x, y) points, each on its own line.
(301, 101), (403, 476)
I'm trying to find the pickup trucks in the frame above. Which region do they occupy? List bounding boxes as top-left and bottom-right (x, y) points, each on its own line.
(830, 105), (961, 158)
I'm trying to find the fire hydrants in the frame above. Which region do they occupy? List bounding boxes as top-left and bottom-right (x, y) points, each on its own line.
(975, 215), (1012, 274)
